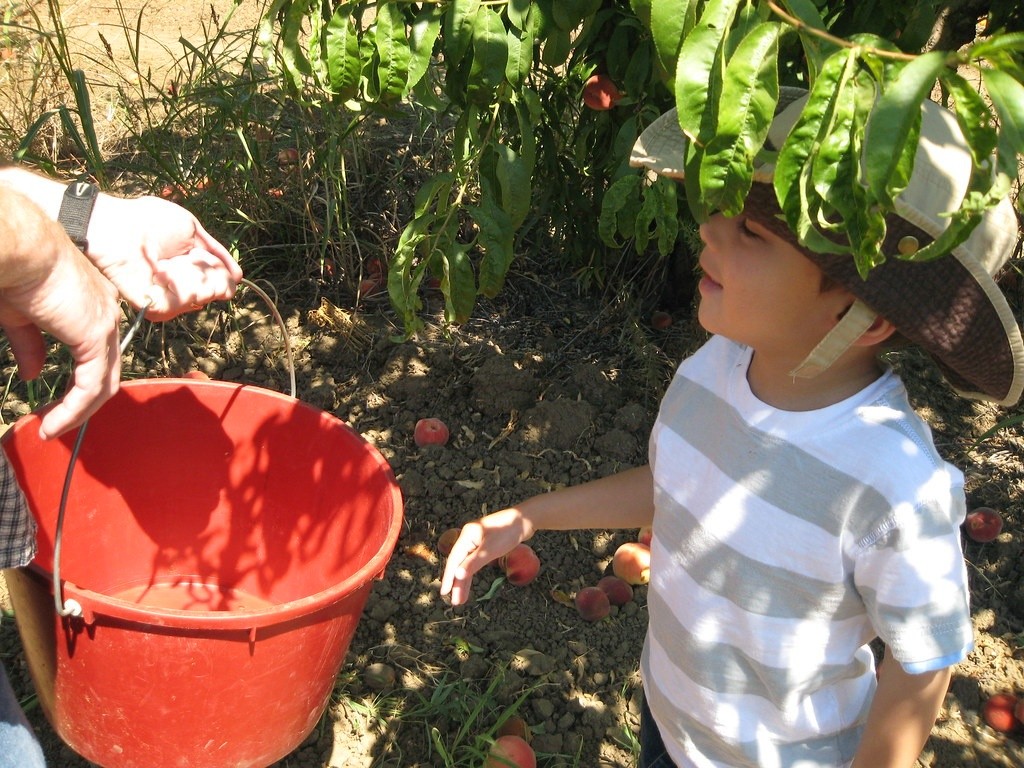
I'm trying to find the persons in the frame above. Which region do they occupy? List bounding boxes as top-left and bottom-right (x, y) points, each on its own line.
(0, 166), (243, 768)
(439, 86), (1016, 768)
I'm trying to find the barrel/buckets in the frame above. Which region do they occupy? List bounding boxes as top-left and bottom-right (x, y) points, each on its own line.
(0, 278), (404, 768)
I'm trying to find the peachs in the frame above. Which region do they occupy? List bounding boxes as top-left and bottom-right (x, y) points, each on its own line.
(157, 76), (1024, 768)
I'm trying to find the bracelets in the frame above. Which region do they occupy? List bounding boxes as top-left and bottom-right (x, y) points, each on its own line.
(55, 180), (100, 256)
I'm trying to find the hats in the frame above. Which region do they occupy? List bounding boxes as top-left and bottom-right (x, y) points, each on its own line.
(627, 77), (1024, 408)
